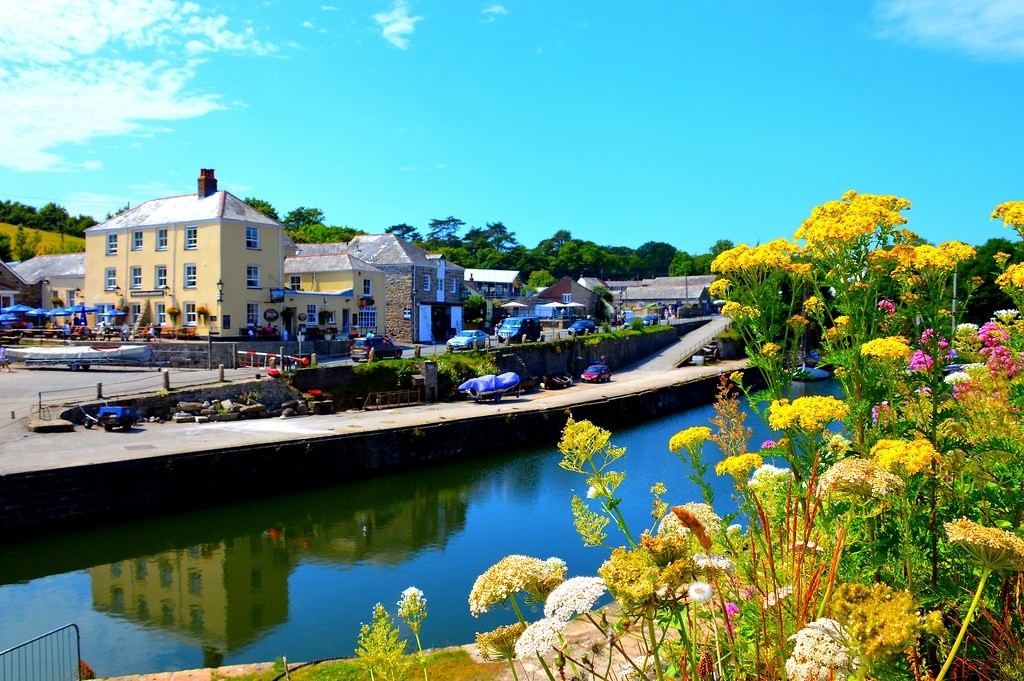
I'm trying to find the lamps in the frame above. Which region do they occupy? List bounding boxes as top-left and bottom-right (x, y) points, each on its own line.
(42, 281), (48, 284)
(112, 286), (120, 291)
(216, 280), (224, 302)
(73, 288), (81, 292)
(159, 285), (170, 291)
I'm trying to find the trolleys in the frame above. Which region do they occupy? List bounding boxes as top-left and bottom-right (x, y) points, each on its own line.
(78, 398), (140, 432)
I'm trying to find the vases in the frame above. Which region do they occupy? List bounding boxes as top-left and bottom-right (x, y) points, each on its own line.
(324, 334), (333, 340)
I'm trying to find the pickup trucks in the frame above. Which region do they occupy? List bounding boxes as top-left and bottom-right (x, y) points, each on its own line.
(568, 320), (595, 336)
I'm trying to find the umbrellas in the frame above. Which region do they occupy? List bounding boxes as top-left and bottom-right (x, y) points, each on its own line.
(102, 309), (128, 317)
(0, 304), (50, 326)
(46, 304), (98, 316)
(542, 301), (586, 307)
(501, 301), (527, 307)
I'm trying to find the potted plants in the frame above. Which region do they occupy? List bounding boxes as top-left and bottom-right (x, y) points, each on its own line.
(296, 329), (305, 342)
(467, 318), (483, 331)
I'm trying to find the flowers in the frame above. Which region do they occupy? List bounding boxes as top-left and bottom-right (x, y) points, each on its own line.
(324, 329), (333, 335)
(320, 311), (333, 321)
(48, 297), (63, 307)
(117, 306), (130, 322)
(280, 310), (293, 322)
(196, 306), (210, 326)
(167, 307), (181, 322)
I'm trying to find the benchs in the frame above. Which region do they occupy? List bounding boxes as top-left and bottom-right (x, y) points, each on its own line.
(160, 327), (196, 339)
(62, 333), (139, 341)
(239, 328), (278, 340)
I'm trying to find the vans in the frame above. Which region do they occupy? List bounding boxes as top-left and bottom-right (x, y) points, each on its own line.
(498, 317), (540, 344)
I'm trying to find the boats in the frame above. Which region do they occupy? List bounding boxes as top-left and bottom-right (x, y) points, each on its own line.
(458, 372), (520, 398)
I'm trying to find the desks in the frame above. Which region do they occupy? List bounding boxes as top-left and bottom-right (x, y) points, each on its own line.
(33, 325), (187, 332)
(10, 328), (64, 340)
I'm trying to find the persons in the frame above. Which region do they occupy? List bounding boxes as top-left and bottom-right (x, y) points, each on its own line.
(45, 314), (91, 339)
(366, 330), (375, 337)
(664, 309), (668, 319)
(351, 328), (359, 334)
(494, 320), (502, 342)
(560, 308), (566, 315)
(21, 320), (37, 338)
(0, 343), (11, 372)
(101, 322), (111, 340)
(121, 321), (130, 341)
(383, 332), (395, 344)
(146, 323), (155, 342)
(248, 323), (259, 341)
(266, 323), (273, 335)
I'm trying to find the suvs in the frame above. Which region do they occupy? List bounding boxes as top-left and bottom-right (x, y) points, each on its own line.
(350, 337), (402, 362)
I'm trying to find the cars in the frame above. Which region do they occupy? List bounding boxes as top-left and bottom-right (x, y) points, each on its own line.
(581, 365), (612, 382)
(624, 315), (658, 329)
(332, 333), (350, 341)
(446, 330), (490, 350)
